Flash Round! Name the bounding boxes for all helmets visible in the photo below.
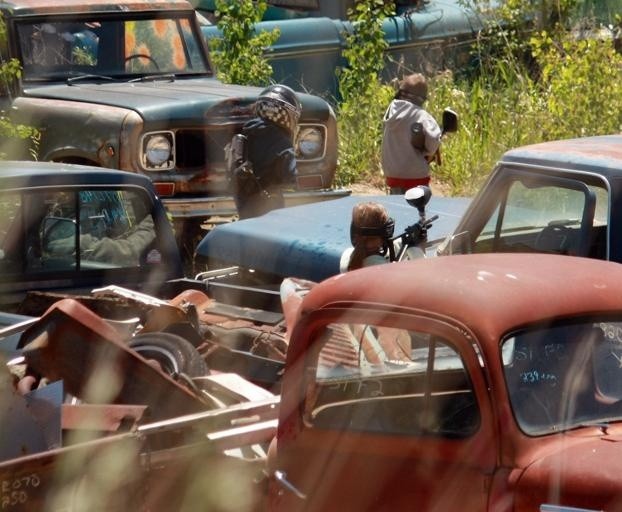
[259,85,303,129]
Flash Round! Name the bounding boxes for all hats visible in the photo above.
[399,72,428,100]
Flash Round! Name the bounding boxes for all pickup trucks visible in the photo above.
[0,3,527,306]
[163,134,622,310]
[1,251,622,512]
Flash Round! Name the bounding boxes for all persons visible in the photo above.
[27,191,173,266]
[381,72,444,194]
[338,202,429,378]
[226,85,303,220]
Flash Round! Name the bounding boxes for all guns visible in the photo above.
[410,108,458,166]
[384,185,438,262]
